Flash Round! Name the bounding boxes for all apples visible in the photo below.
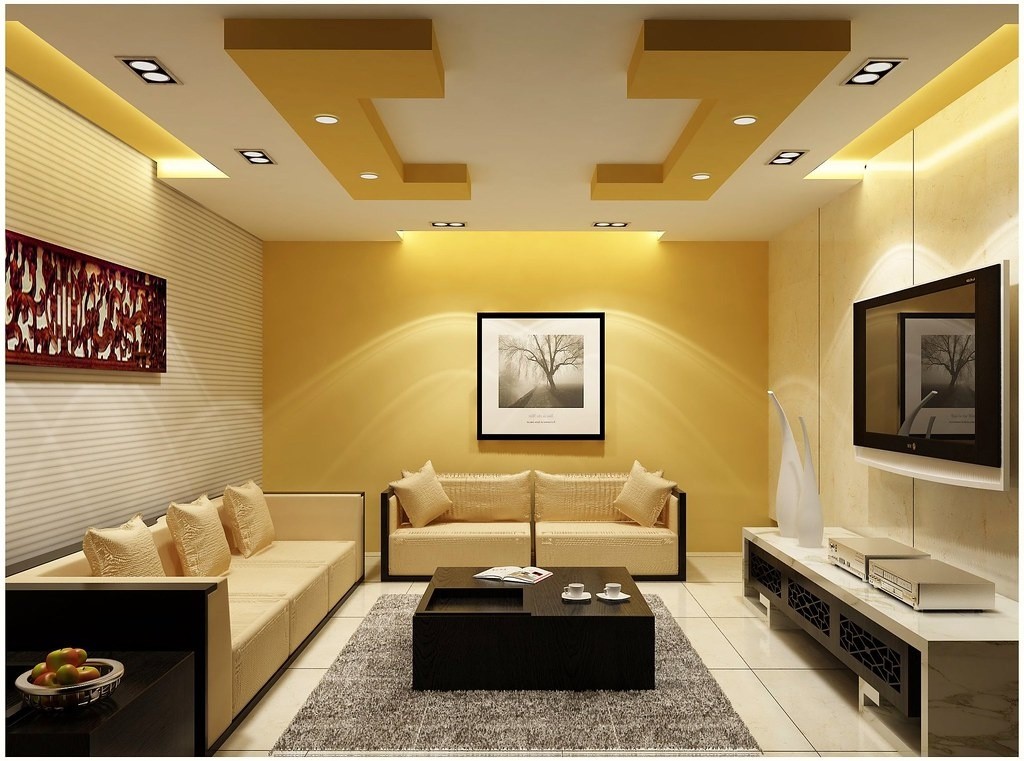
[29,646,100,704]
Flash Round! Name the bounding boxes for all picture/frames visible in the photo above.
[898,312,976,440]
[477,312,606,441]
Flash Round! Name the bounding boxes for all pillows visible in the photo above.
[613,460,678,528]
[166,489,232,577]
[388,460,452,528]
[222,478,274,558]
[82,512,166,577]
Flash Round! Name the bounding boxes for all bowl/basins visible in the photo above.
[15,658,125,709]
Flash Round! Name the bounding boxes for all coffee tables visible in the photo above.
[413,567,656,692]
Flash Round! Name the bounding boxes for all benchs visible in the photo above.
[380,470,687,582]
[5,491,366,757]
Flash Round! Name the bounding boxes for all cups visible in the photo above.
[564,583,584,598]
[604,583,622,597]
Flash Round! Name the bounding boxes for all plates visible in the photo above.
[561,591,591,600]
[595,592,632,601]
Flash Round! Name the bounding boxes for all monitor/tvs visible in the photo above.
[855,268,1001,468]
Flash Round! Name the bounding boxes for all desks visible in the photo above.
[6,650,195,756]
[741,526,1019,757]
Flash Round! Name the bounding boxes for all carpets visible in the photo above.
[267,593,763,756]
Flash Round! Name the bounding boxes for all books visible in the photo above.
[473,566,553,584]
